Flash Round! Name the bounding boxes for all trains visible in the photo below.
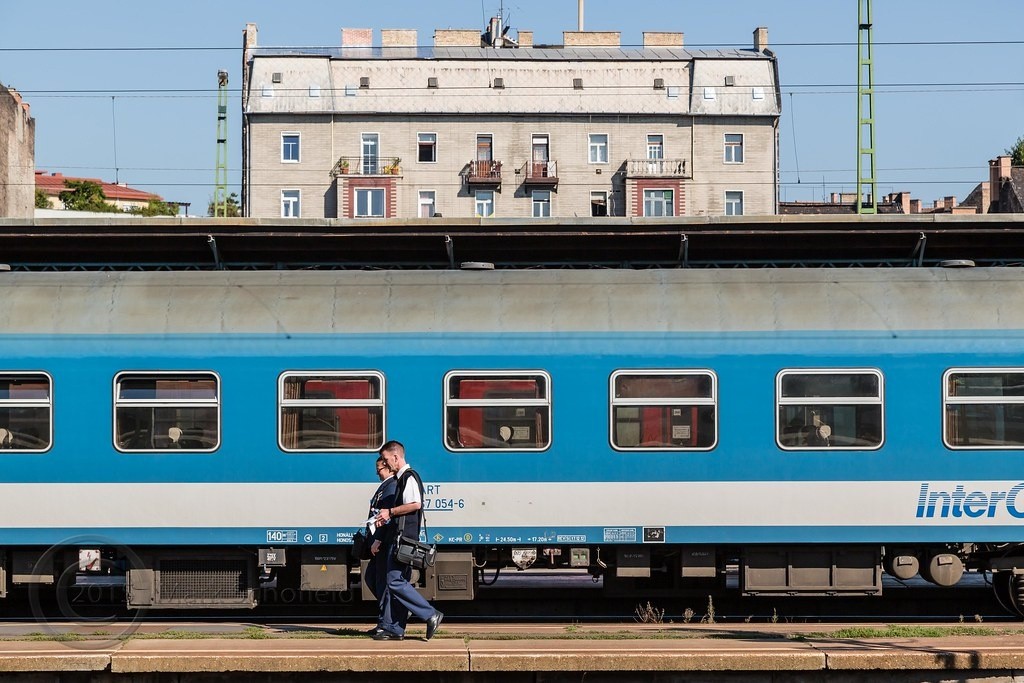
[0,209,1023,624]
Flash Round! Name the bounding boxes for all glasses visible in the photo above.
[376,466,388,474]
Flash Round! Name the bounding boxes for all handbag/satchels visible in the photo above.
[396,535,437,570]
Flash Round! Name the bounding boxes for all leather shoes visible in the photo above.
[426,610,444,640]
[372,631,404,641]
[406,609,412,620]
[367,626,384,636]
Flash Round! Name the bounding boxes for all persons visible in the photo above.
[363,441,443,641]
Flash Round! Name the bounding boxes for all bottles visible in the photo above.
[371,506,391,524]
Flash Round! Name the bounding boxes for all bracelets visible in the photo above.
[389,508,393,517]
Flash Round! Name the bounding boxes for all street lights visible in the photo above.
[213,69,230,218]
